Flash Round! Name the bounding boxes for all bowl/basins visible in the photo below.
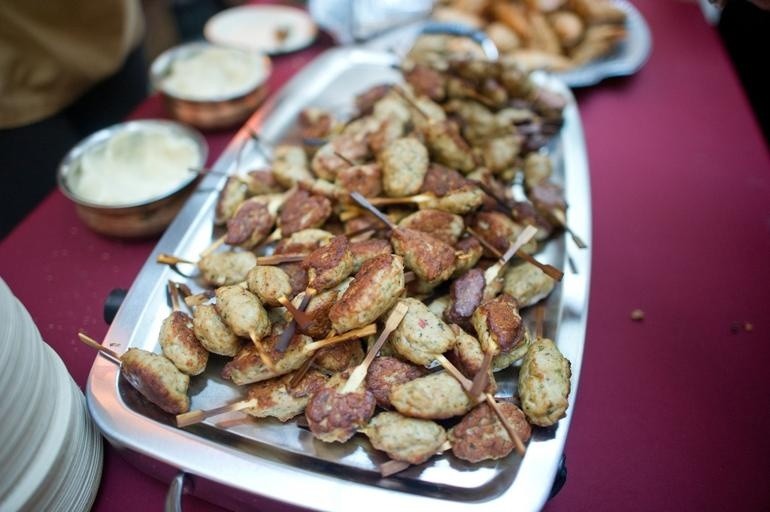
[149,39,273,128]
[57,118,212,242]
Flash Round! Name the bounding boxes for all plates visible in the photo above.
[1,277,104,510]
[201,1,322,51]
[428,1,655,90]
